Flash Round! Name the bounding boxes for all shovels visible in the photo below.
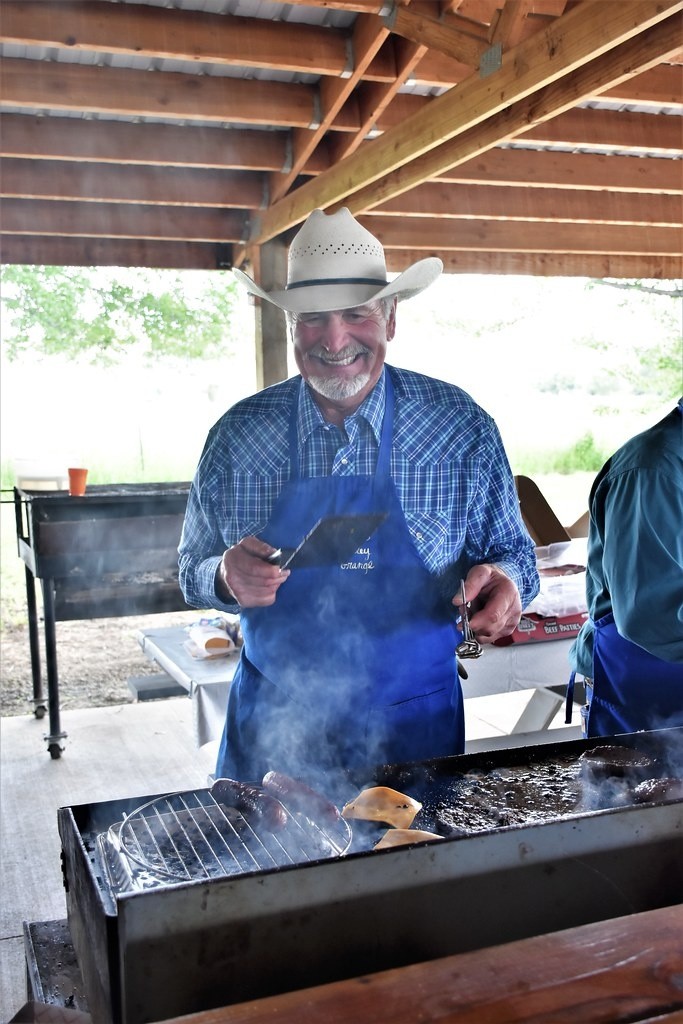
[269,512,389,571]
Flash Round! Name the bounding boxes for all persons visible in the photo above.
[177,208,541,778]
[570,397,683,737]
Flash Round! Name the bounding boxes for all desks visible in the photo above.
[136,618,587,788]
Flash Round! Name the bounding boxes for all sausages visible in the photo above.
[262,771,341,825]
[211,777,287,833]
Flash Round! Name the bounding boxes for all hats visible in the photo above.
[232,206,445,314]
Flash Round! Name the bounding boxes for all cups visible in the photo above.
[68,468,88,496]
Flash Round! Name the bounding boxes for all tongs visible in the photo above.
[455,579,484,659]
